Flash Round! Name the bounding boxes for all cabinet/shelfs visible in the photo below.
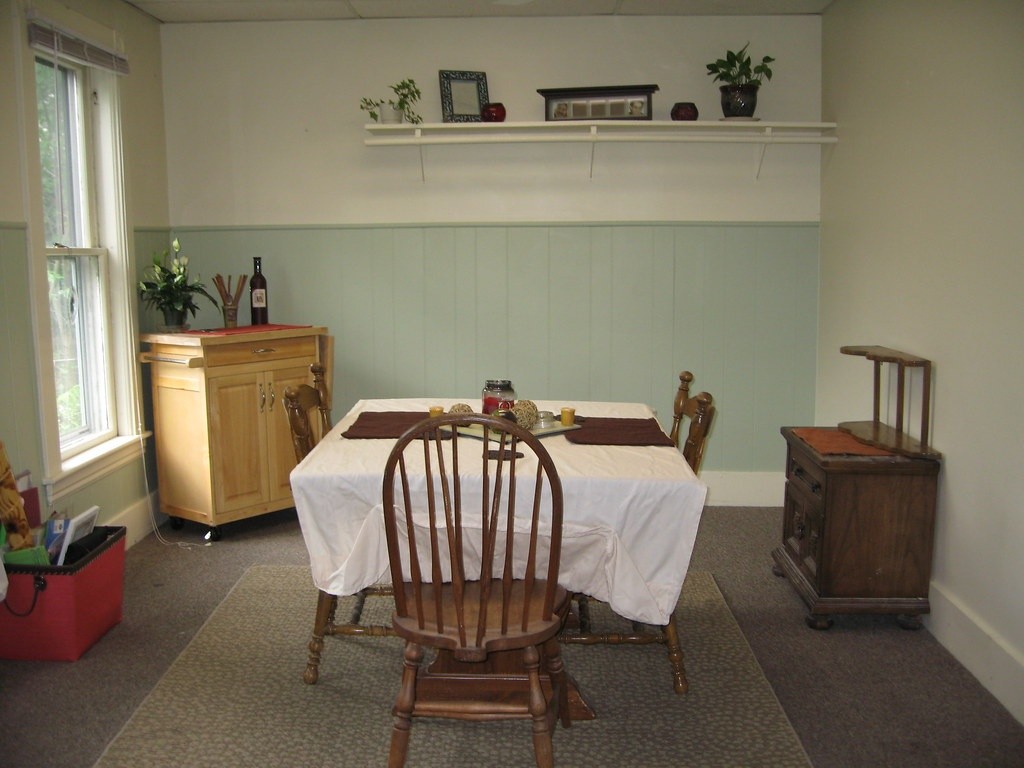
[139,324,333,539]
[838,345,942,461]
[772,426,940,629]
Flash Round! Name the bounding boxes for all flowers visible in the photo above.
[140,236,217,318]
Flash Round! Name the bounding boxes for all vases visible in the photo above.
[164,302,186,327]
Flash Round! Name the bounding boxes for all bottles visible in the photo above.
[249,256,268,325]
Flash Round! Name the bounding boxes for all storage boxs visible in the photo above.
[1,512,126,663]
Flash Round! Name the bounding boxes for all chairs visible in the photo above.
[283,364,402,687]
[382,411,571,768]
[556,370,715,694]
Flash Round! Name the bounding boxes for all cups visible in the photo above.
[429,406,443,418]
[561,407,575,426]
[222,306,238,329]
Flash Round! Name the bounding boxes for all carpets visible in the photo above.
[92,563,816,768]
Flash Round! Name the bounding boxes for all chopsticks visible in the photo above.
[212,273,247,307]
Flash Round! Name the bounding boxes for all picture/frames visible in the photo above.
[438,68,491,122]
[57,505,101,565]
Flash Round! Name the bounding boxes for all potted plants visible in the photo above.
[359,76,423,126]
[708,41,776,120]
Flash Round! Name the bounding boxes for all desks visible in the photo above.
[290,396,708,718]
